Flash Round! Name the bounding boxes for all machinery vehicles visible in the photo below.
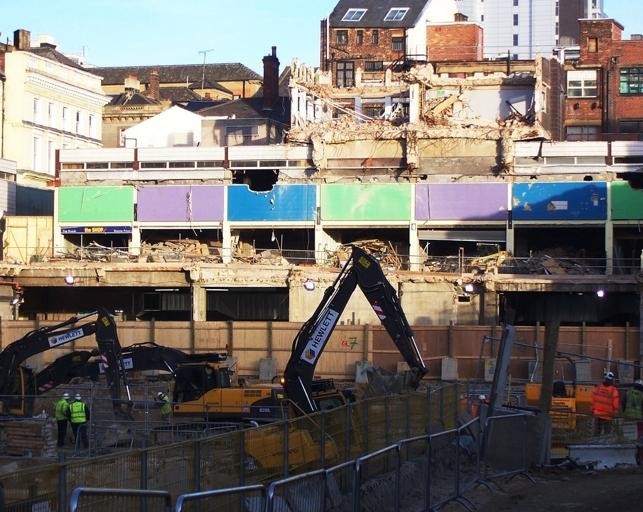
[0,246,289,424]
[234,244,430,480]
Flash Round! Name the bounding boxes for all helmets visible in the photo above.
[74,394,80,400]
[63,393,71,400]
[157,391,163,396]
[634,379,643,387]
[605,371,615,380]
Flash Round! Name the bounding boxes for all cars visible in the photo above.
[425,251,590,276]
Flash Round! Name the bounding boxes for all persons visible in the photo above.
[469,393,488,419]
[54,392,70,447]
[67,392,90,449]
[151,390,172,426]
[458,393,469,415]
[621,378,643,422]
[588,370,620,442]
